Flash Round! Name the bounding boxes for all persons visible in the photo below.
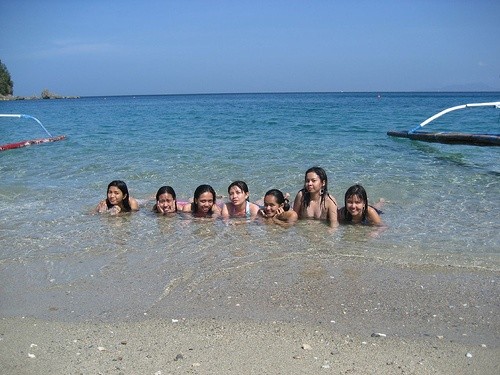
[153,185,187,219]
[337,184,387,239]
[293,166,338,236]
[257,188,299,228]
[95,180,156,216]
[221,180,264,226]
[183,184,222,223]
